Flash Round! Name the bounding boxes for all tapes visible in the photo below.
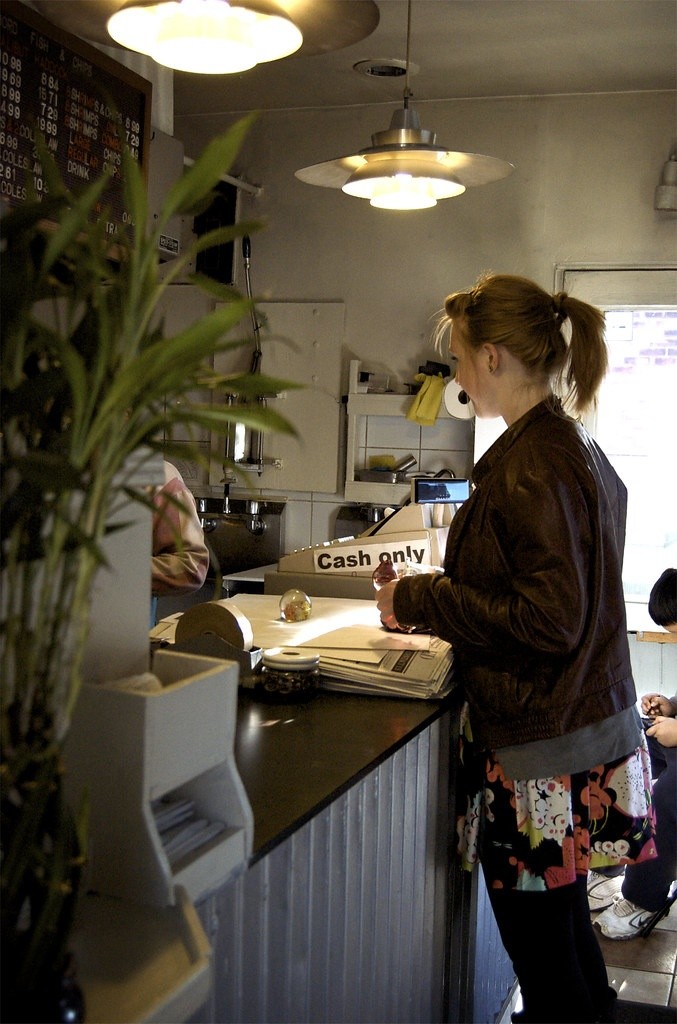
[173,599,254,652]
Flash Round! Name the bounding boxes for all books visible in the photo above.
[147,594,456,700]
[148,780,229,869]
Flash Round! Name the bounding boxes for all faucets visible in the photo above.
[195,498,218,532]
[246,500,267,536]
[220,477,239,515]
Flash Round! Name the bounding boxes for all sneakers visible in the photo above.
[591,892,665,941]
[587,869,628,911]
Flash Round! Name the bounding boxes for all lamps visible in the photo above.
[33,0,380,74]
[295,0,516,211]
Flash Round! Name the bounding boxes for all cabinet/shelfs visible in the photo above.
[345,360,473,505]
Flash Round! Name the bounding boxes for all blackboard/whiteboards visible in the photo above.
[1,0,153,265]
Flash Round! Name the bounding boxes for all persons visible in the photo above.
[375,276,657,1024]
[112,400,210,628]
[585,568,677,939]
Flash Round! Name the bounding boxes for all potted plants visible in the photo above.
[0,108,338,1024]
